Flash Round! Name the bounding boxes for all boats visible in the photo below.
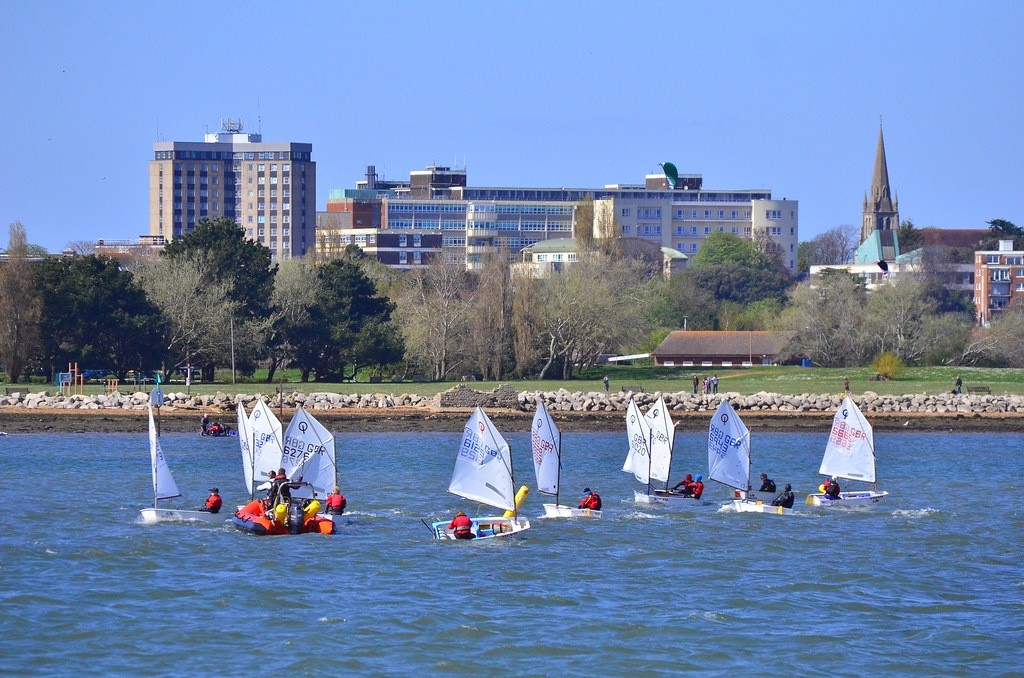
[232,479,337,536]
[200,422,239,437]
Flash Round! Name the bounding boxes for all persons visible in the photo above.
[448,511,476,540]
[693,375,699,394]
[325,486,346,515]
[603,375,609,391]
[256,468,303,520]
[759,474,776,492]
[578,488,601,510]
[200,488,222,513]
[844,378,849,392]
[672,474,704,500]
[201,414,208,434]
[702,374,719,393]
[955,376,962,394]
[772,484,794,508]
[824,478,840,500]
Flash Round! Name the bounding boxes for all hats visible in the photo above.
[695,475,702,480]
[583,488,591,495]
[686,474,692,480]
[209,488,219,493]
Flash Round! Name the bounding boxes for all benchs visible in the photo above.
[966,386,992,395]
[275,387,294,395]
[622,386,644,393]
[6,387,30,396]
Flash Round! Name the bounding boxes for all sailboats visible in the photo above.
[531,400,604,519]
[420,402,531,541]
[621,395,704,507]
[236,399,338,521]
[805,395,889,507]
[707,397,794,515]
[139,402,234,523]
